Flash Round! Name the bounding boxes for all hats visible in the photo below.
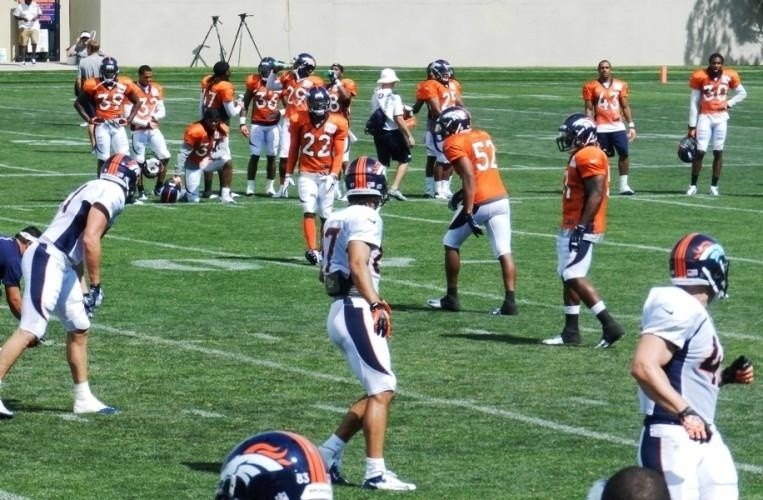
[203,107,223,122]
[14,224,38,243]
[213,61,230,75]
[376,68,400,83]
[80,32,90,38]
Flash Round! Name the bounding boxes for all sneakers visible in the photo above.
[449,176,454,196]
[387,185,407,201]
[441,180,452,199]
[272,185,289,198]
[362,469,416,491]
[126,196,143,204]
[423,177,435,199]
[318,460,352,485]
[305,249,320,265]
[427,296,460,311]
[489,301,519,316]
[151,180,165,195]
[686,184,697,195]
[202,192,219,199]
[230,191,239,198]
[0,397,13,416]
[138,185,149,200]
[265,190,277,196]
[538,329,582,345]
[216,195,238,204]
[431,181,448,199]
[590,324,626,349]
[619,186,634,194]
[709,185,719,195]
[246,191,256,195]
[73,396,122,414]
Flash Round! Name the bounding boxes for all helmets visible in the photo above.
[434,107,471,142]
[345,156,390,207]
[258,57,277,82]
[160,177,181,202]
[100,153,141,204]
[307,86,331,117]
[670,232,729,300]
[556,113,598,152]
[290,53,316,79]
[99,57,120,90]
[430,59,454,89]
[143,154,164,179]
[678,136,697,163]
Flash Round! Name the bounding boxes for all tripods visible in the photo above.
[219,46,227,63]
[227,13,264,70]
[189,16,226,69]
[190,46,210,69]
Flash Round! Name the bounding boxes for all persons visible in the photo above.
[74,57,147,205]
[413,59,464,200]
[167,109,236,203]
[544,113,627,350]
[0,225,45,346]
[283,86,349,266]
[73,40,105,153]
[13,0,43,65]
[200,61,244,204]
[66,32,106,67]
[266,53,325,196]
[588,467,674,500]
[366,68,415,201]
[582,59,636,195]
[325,64,357,201]
[426,108,518,315]
[124,65,170,200]
[0,155,142,417]
[689,52,747,195]
[240,57,282,196]
[319,158,418,491]
[631,232,755,499]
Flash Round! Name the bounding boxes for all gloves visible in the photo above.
[569,225,586,252]
[673,405,713,444]
[371,298,392,338]
[626,122,637,142]
[448,189,465,210]
[319,173,338,191]
[721,357,754,384]
[83,293,94,319]
[89,282,104,311]
[466,213,484,237]
[408,136,415,148]
[89,116,104,125]
[112,118,128,128]
[282,173,295,197]
